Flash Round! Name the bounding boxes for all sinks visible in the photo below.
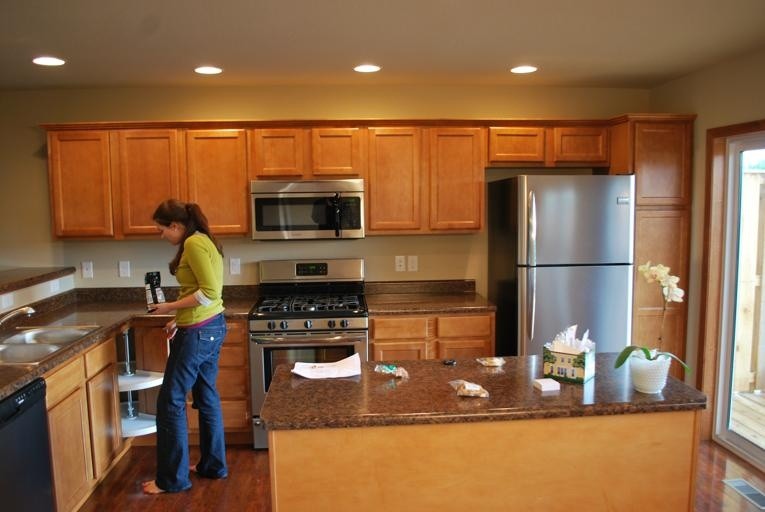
[2,324,104,344]
[0,345,66,366]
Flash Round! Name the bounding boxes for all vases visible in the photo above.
[629,352,671,395]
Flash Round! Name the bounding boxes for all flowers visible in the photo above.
[613,257,694,377]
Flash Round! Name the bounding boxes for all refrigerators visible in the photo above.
[485,171,636,356]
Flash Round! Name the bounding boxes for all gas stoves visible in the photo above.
[248,284,368,330]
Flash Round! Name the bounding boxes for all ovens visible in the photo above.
[247,331,368,451]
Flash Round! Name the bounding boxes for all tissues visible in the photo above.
[542,324,597,386]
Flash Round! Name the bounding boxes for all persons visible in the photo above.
[141,197,227,495]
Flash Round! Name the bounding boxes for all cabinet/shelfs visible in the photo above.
[243,120,362,178]
[601,112,697,382]
[39,119,252,242]
[370,312,496,362]
[134,320,252,446]
[43,337,124,512]
[354,119,487,236]
[487,119,608,168]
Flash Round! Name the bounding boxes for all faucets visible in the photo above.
[0,306,36,327]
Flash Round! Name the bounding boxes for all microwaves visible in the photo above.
[250,180,366,240]
